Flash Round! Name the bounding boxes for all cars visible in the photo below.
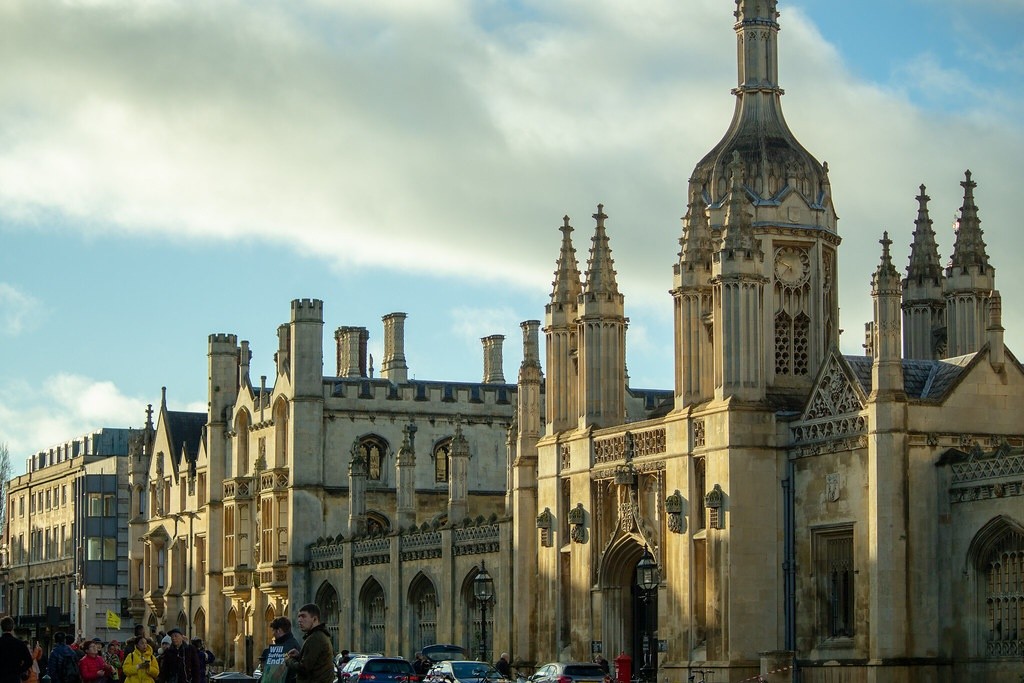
[524,662,616,683]
[332,644,511,683]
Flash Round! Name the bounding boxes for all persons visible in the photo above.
[594,655,610,675]
[496,653,512,681]
[0,616,127,683]
[260,616,301,658]
[338,649,351,666]
[284,603,335,683]
[120,625,215,683]
[411,652,425,674]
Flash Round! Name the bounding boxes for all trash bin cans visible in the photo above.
[757,650,795,683]
[208,671,259,683]
[614,655,632,683]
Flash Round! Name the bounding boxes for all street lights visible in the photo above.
[636,543,658,683]
[473,559,494,661]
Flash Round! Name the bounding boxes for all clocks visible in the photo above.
[773,245,811,289]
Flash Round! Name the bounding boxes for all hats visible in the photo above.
[257,649,270,659]
[167,628,182,635]
[110,640,120,647]
[92,638,105,648]
[158,634,173,655]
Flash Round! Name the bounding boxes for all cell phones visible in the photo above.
[145,661,150,663]
[37,641,39,648]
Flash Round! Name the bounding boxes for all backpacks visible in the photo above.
[54,648,79,682]
